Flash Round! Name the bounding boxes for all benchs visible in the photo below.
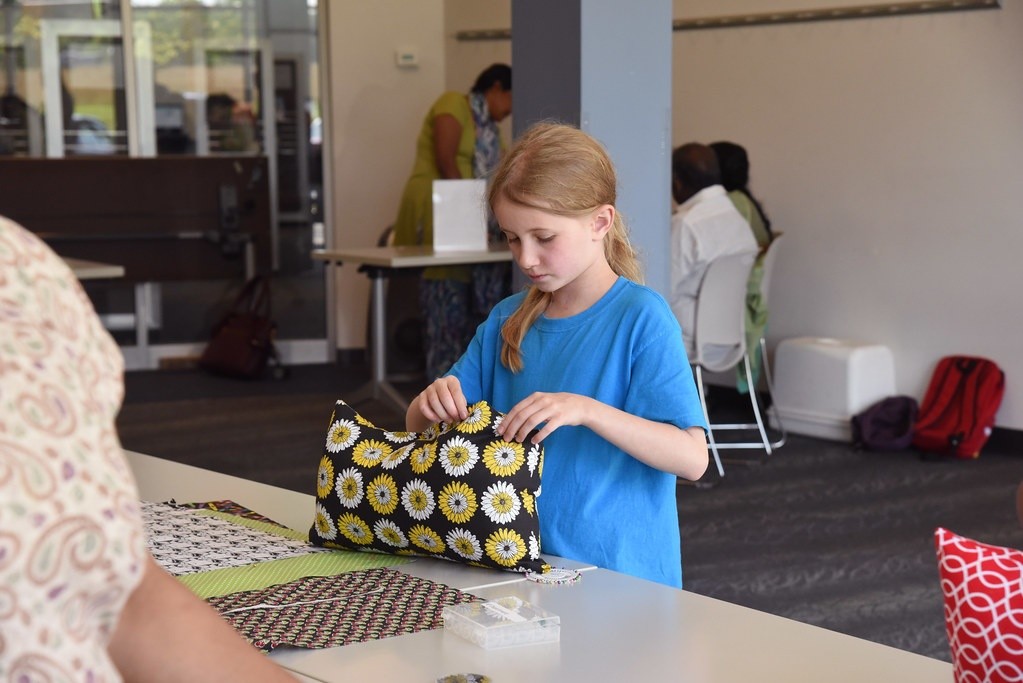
[0,155,266,370]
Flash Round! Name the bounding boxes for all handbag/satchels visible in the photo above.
[852,395,920,453]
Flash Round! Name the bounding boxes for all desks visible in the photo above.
[58,255,126,278]
[311,240,514,420]
[124,450,954,683]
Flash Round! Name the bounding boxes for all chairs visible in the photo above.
[682,230,787,489]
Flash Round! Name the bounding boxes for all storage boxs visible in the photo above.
[765,336,897,441]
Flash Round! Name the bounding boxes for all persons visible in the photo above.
[0,214,298,683]
[394,61,512,393]
[406,123,708,587]
[673,142,756,359]
[711,141,772,348]
[207,94,236,120]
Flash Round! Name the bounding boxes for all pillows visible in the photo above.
[308,399,550,575]
[934,527,1023,683]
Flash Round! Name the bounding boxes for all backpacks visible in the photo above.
[200,270,291,383]
[911,357,1004,459]
